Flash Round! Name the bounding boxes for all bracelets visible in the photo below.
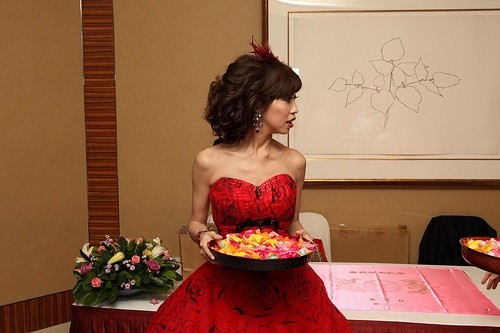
[196,231,209,247]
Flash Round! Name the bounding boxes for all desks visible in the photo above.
[69,262,500,333]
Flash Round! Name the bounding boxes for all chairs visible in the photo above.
[207,212,332,261]
[418,215,497,265]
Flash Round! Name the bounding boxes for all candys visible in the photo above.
[209,228,316,259]
[466,238,500,257]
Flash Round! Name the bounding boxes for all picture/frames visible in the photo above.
[261,0,500,191]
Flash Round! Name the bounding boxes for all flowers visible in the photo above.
[71,234,185,307]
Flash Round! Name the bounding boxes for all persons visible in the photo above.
[139,36,355,333]
[481,271,500,291]
